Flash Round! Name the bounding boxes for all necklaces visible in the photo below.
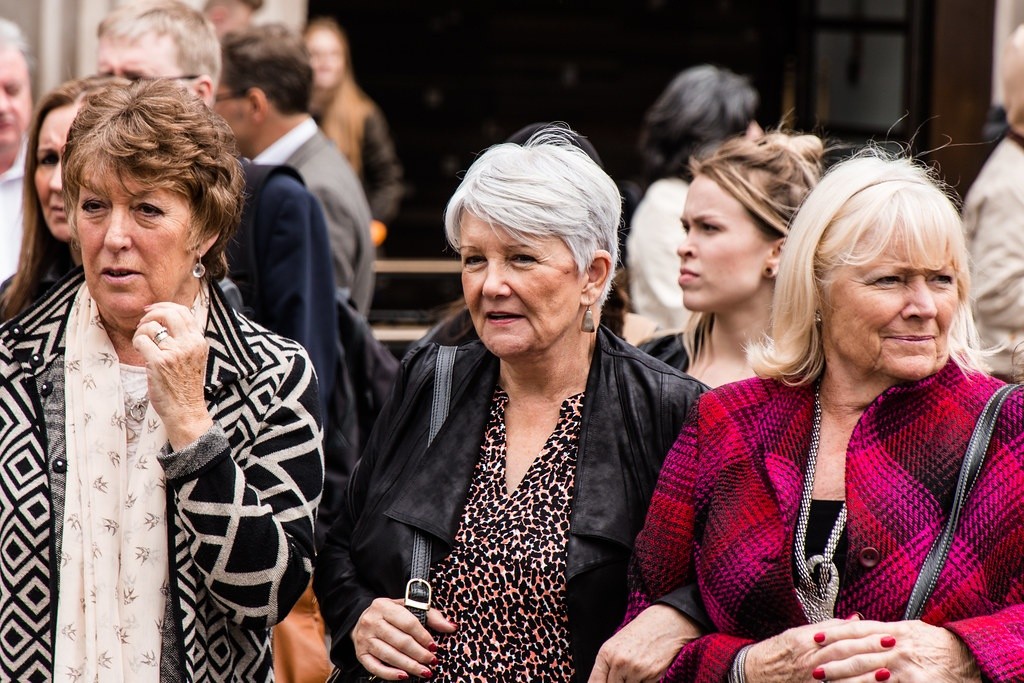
[793,380,848,625]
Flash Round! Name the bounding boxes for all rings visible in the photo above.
[154,327,168,343]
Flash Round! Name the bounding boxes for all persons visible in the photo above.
[313,122,713,683]
[632,120,825,392]
[0,0,408,683]
[961,28,1024,383]
[616,145,1023,683]
[617,63,767,339]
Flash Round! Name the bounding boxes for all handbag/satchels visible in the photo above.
[325,345,458,683]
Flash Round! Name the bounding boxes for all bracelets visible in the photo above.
[727,643,754,683]
